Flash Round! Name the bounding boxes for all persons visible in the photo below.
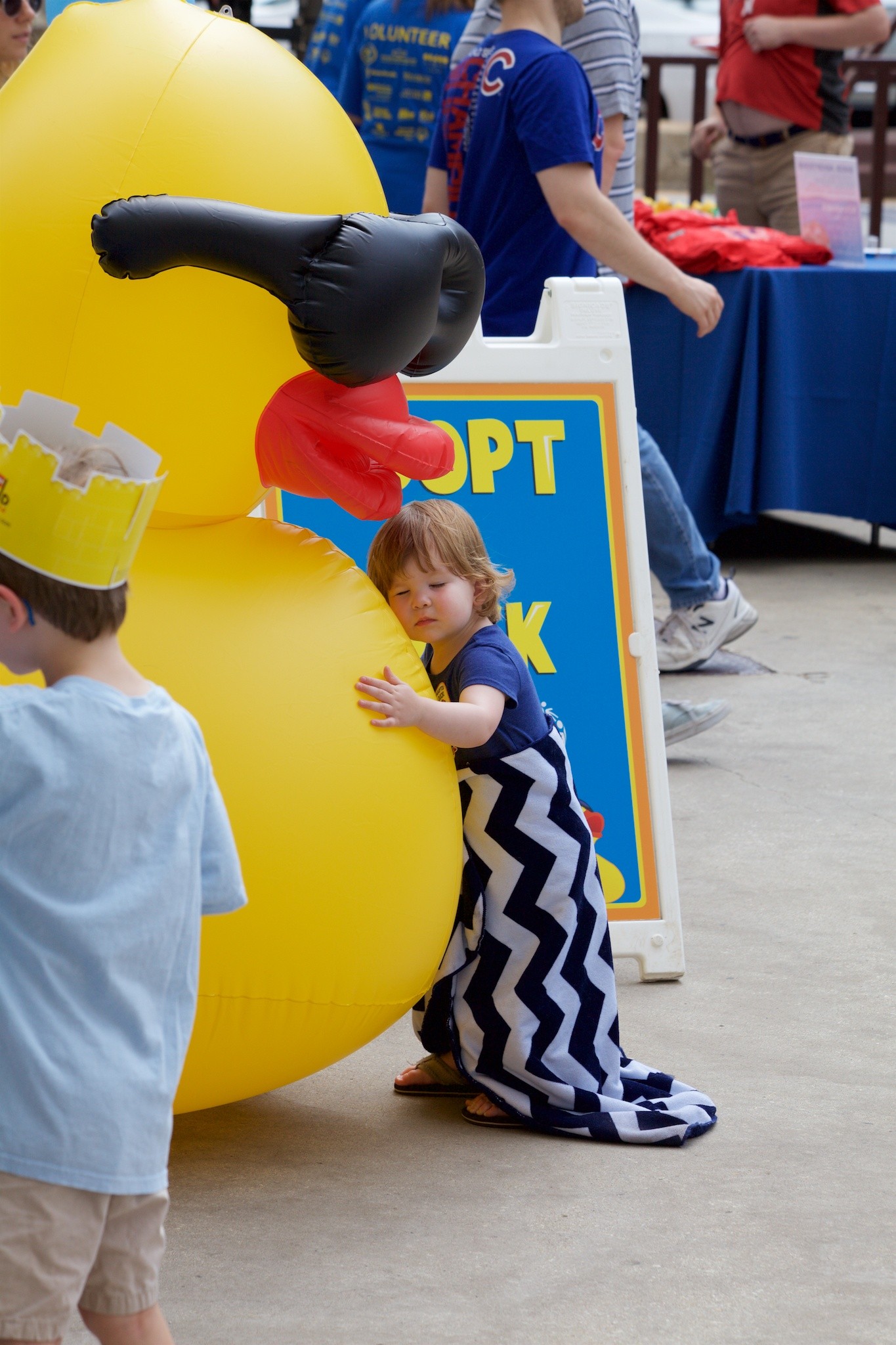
[419,0,733,746]
[448,0,760,671]
[0,391,249,1345]
[353,500,718,1145]
[689,0,890,235]
[1,0,472,265]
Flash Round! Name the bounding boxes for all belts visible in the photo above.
[725,120,805,148]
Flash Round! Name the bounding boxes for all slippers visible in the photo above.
[460,1092,521,1125]
[393,1053,479,1095]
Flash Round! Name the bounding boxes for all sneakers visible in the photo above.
[659,698,733,747]
[654,569,758,672]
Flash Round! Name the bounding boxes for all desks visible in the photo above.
[622,253,896,555]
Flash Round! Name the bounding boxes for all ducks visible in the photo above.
[0,1,465,1113]
